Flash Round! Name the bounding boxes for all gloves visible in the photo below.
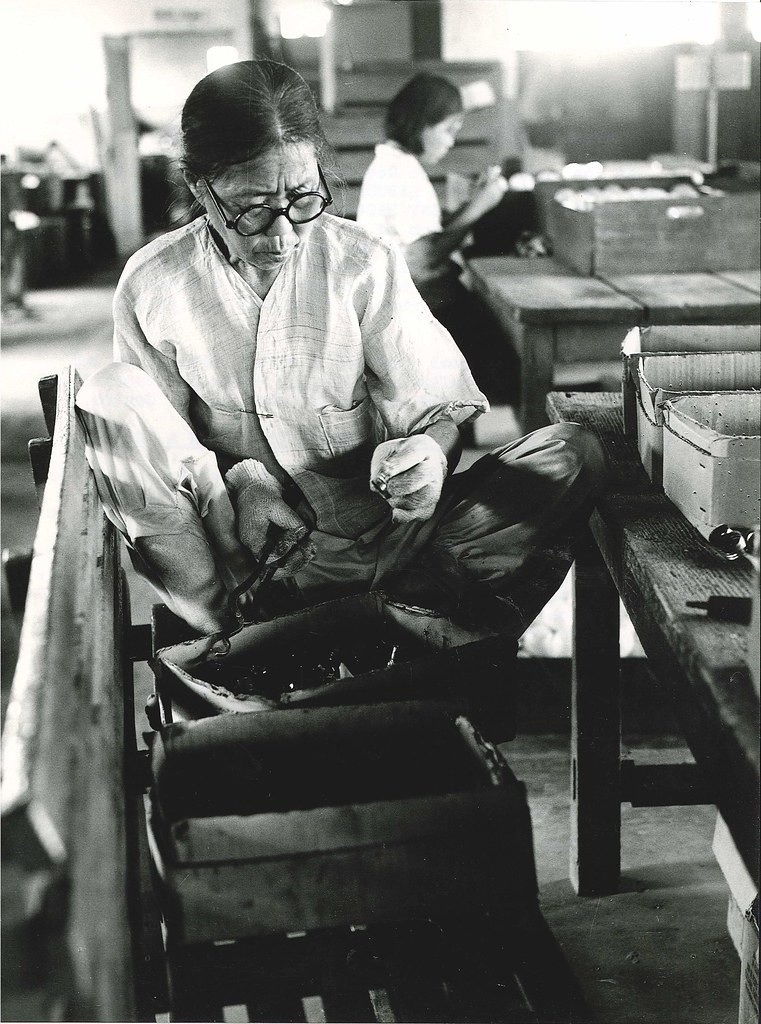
[370,433,450,523]
[224,459,317,581]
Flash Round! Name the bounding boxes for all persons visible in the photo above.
[109,61,605,741]
[356,71,518,410]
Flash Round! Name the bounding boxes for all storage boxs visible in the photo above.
[548,190,760,278]
[153,589,518,741]
[531,175,692,256]
[618,324,761,438]
[661,393,761,541]
[635,352,760,488]
[147,696,539,947]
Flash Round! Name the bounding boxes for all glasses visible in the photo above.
[203,162,335,237]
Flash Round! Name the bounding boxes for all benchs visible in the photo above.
[0,364,592,1023]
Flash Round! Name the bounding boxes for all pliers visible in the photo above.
[208,518,312,656]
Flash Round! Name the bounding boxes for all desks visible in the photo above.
[542,391,761,899]
[467,257,761,439]
